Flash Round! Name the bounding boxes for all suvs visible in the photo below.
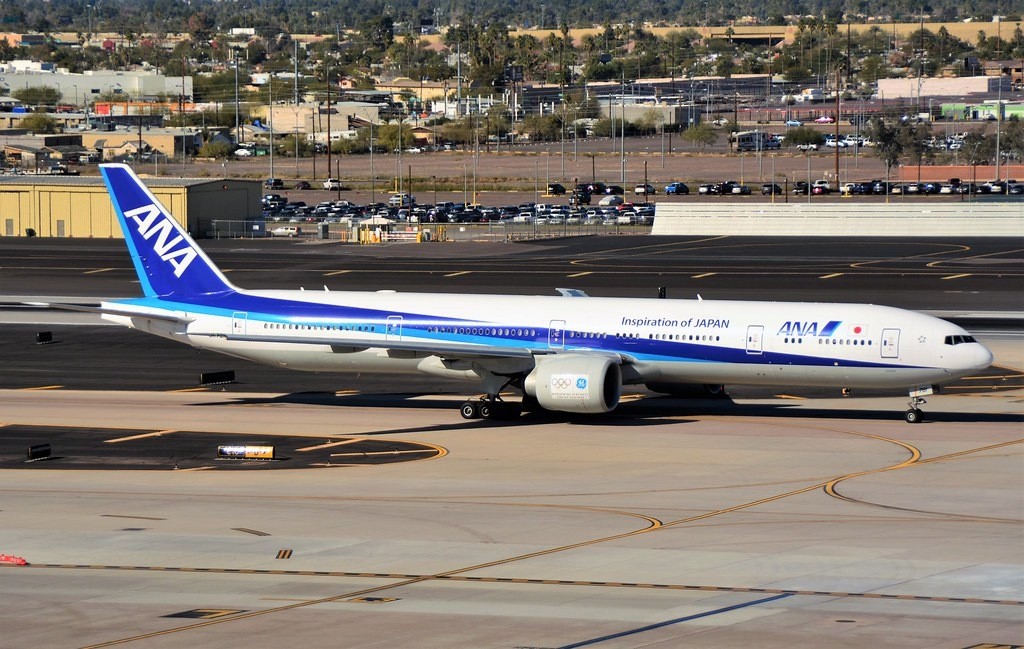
[849,113,871,125]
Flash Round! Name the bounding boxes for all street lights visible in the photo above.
[772,84,801,137]
[678,88,708,147]
[384,98,416,194]
[559,98,592,161]
[281,99,312,180]
[736,91,774,166]
[232,45,244,148]
[362,106,387,174]
[545,106,582,182]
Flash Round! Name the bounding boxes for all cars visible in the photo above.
[263,175,1020,231]
[786,120,803,127]
[901,115,918,124]
[814,116,834,125]
[723,128,1024,161]
[404,140,456,154]
[713,118,729,126]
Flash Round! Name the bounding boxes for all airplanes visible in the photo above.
[21,160,994,425]
